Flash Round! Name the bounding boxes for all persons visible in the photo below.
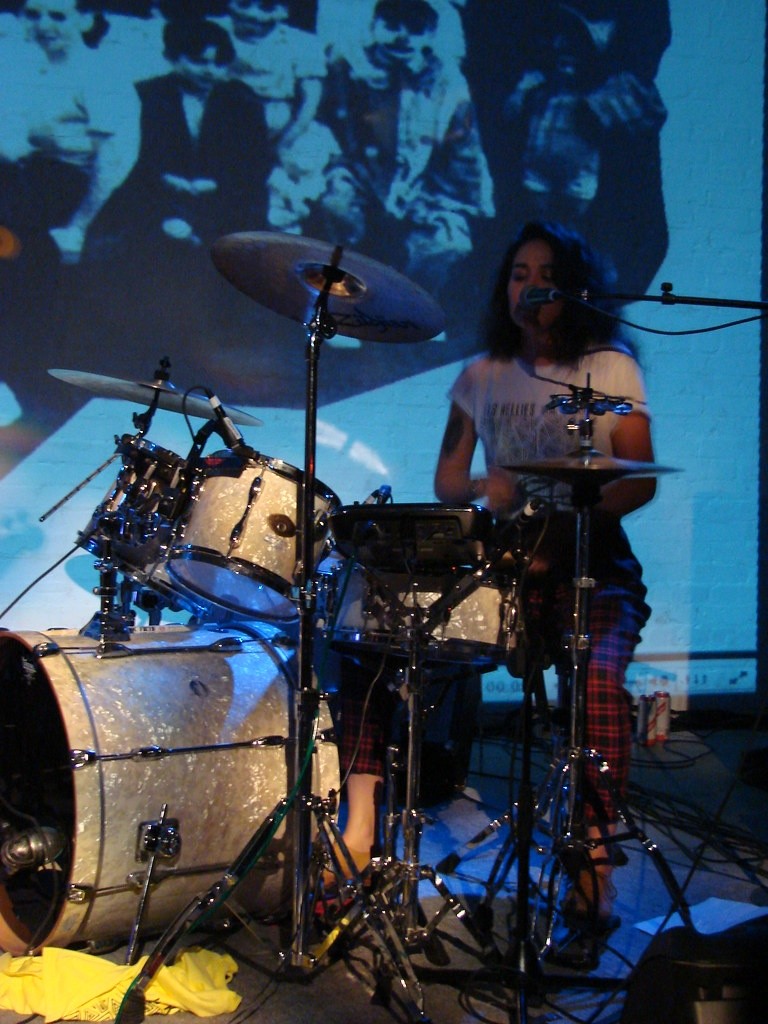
[0,1,672,407]
[304,220,655,919]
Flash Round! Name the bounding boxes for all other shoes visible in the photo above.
[560,870,616,920]
[322,845,378,887]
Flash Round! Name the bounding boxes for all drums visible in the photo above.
[330,548,511,668]
[161,445,344,622]
[72,433,182,594]
[1,619,345,948]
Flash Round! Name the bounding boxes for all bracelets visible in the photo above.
[468,479,480,497]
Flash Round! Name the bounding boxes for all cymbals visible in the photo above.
[210,228,444,346]
[497,449,685,486]
[46,366,263,427]
